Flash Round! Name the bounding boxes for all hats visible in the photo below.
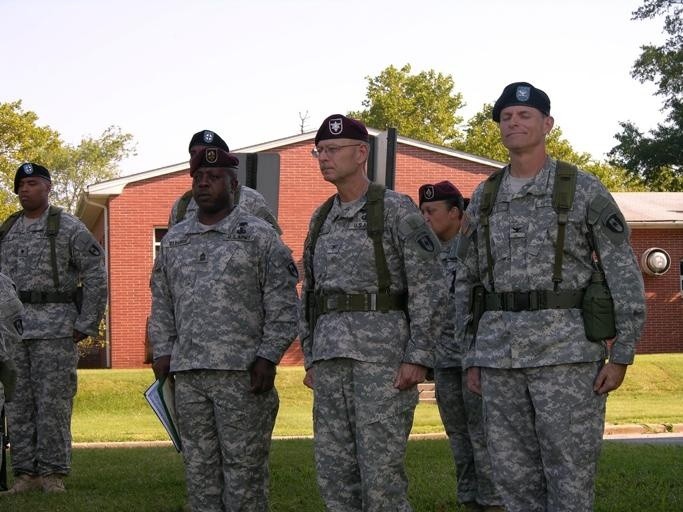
[191,149,240,172]
[15,163,52,191]
[316,114,368,141]
[188,130,228,150]
[419,181,463,203]
[493,82,550,119]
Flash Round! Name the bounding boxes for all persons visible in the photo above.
[417,178,488,510]
[452,81,646,512]
[150,145,298,512]
[0,161,108,496]
[167,129,284,237]
[0,272,28,470]
[296,112,443,512]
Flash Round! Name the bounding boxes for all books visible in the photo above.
[143,371,182,455]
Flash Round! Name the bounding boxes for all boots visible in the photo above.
[1,473,40,497]
[40,474,67,496]
